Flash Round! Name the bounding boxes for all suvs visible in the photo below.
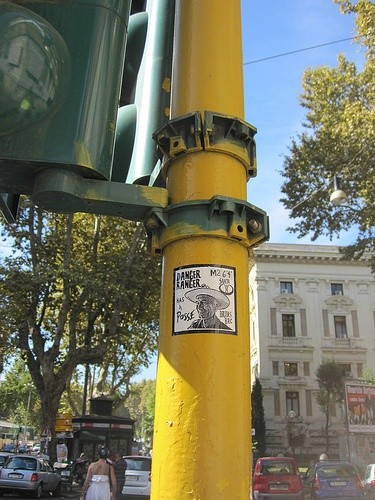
[116,455,151,500]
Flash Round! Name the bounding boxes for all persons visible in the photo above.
[80,447,117,500]
[111,451,127,497]
[76,453,87,486]
[2,443,33,455]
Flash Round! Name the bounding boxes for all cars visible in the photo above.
[33,444,41,451]
[311,459,375,500]
[253,457,306,500]
[0,451,62,499]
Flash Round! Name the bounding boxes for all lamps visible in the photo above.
[330,175,347,205]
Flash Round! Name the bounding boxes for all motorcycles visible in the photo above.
[66,451,98,487]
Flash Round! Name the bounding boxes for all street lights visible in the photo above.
[22,382,38,439]
